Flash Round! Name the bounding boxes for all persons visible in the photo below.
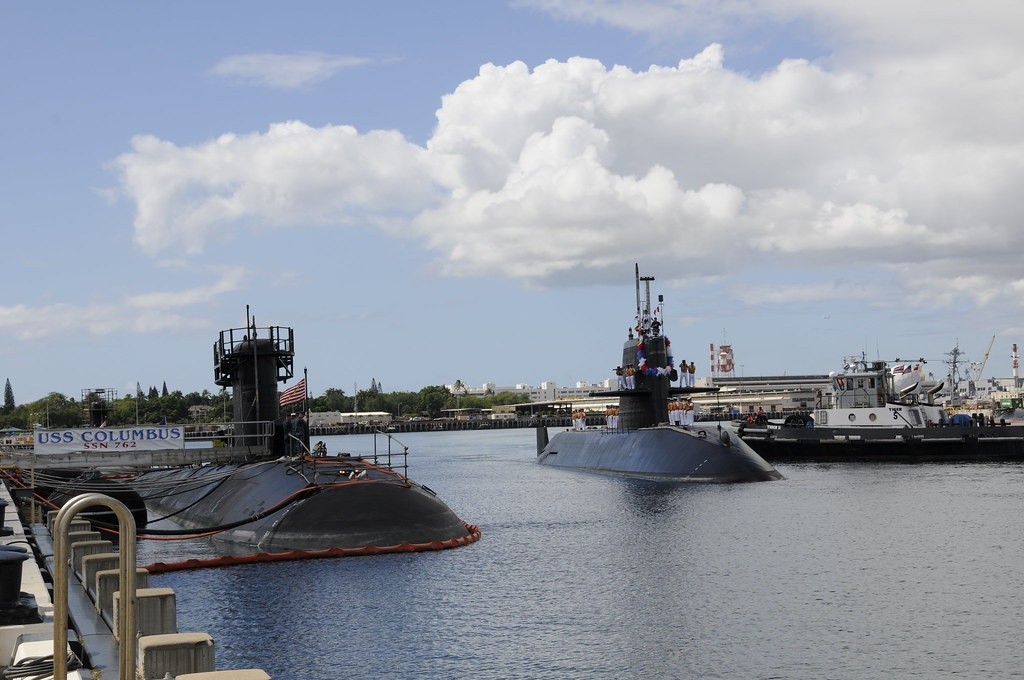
[747,415,753,424]
[625,364,631,390]
[757,407,764,414]
[295,411,308,456]
[651,317,661,336]
[667,397,694,429]
[630,363,636,389]
[291,413,298,458]
[679,360,689,388]
[605,405,620,430]
[642,310,655,334]
[613,367,628,391]
[572,408,586,431]
[282,414,293,457]
[688,362,696,388]
[314,441,327,458]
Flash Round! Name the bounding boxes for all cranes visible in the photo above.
[976,334,995,379]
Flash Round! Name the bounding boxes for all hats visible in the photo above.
[285,413,291,416]
[666,395,692,401]
[291,412,297,415]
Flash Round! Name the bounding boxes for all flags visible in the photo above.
[280,378,305,406]
[100,420,107,429]
[914,366,918,370]
[893,365,904,374]
[902,365,911,375]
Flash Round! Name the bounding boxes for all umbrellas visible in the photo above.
[1,426,25,433]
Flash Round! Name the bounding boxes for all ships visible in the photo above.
[729,332,1024,462]
[532,262,785,484]
[119,303,470,559]
